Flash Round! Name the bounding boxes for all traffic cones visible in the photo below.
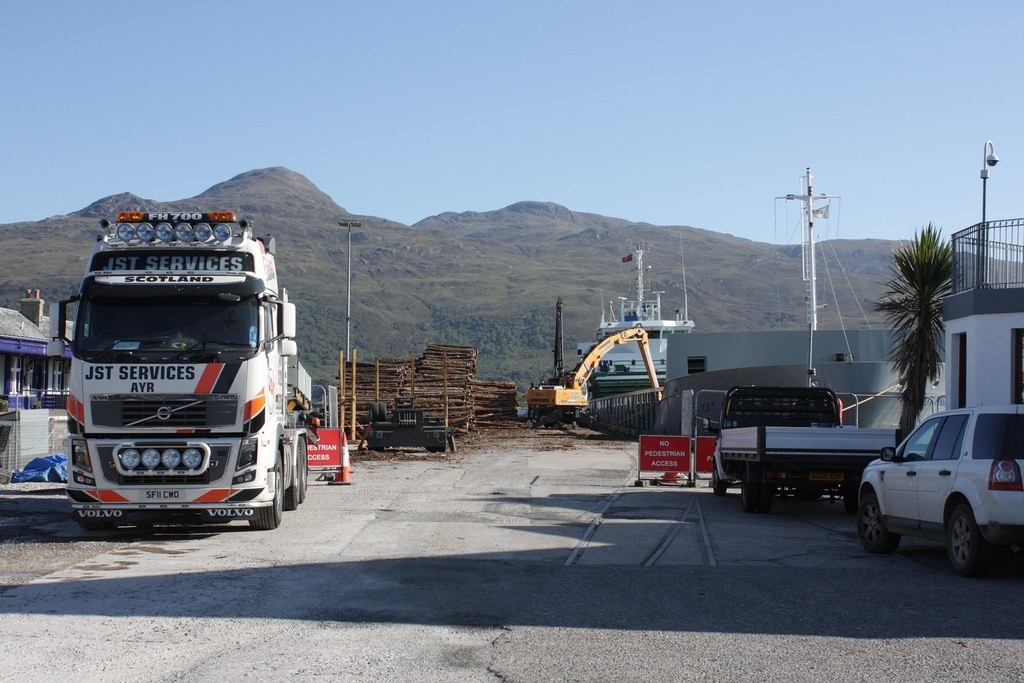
[328,432,355,485]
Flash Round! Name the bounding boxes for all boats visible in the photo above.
[577,238,696,429]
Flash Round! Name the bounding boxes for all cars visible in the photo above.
[856,404,1024,577]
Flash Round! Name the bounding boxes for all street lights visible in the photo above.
[338,219,362,443]
[979,140,1000,289]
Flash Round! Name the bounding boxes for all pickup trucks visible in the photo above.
[702,385,905,514]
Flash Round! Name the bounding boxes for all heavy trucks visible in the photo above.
[47,211,320,531]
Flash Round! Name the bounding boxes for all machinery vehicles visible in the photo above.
[527,328,663,428]
